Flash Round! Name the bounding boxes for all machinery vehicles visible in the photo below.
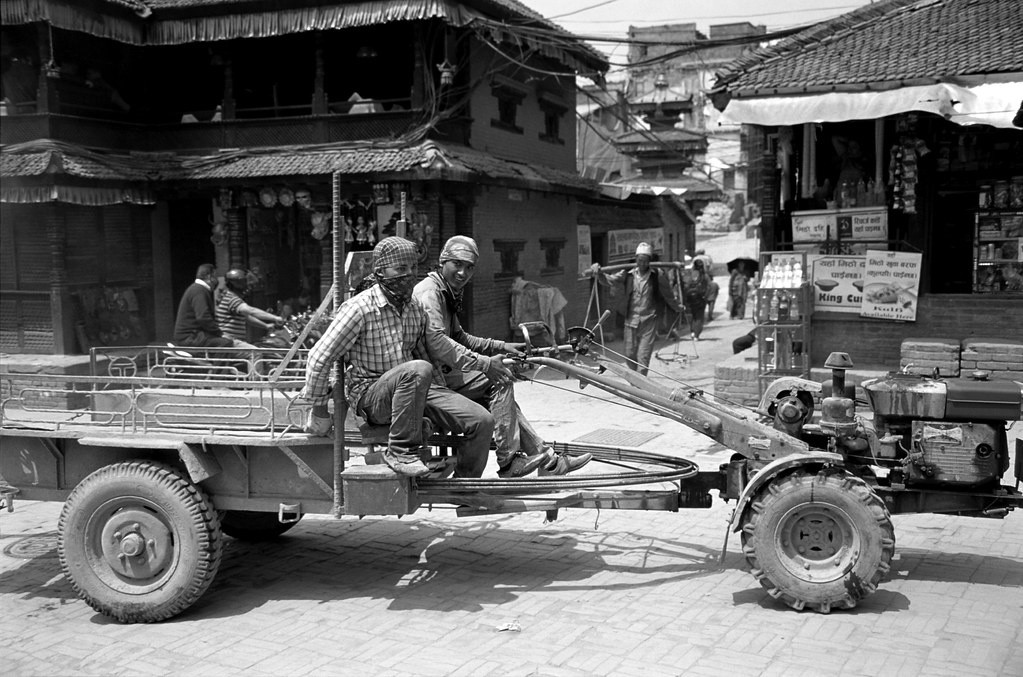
[0,171,1023,627]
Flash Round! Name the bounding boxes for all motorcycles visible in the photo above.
[162,305,320,391]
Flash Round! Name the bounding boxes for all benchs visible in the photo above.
[360,417,458,467]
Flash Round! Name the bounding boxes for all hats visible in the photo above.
[440,235,480,266]
[635,242,654,260]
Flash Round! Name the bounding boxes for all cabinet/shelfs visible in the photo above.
[792,205,887,256]
[756,251,814,402]
[973,212,1023,294]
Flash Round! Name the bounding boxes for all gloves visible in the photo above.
[307,413,335,439]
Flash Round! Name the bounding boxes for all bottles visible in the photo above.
[842,177,876,208]
[758,261,803,322]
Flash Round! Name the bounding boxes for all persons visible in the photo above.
[832,135,874,202]
[686,248,748,341]
[300,236,502,512]
[411,234,593,479]
[215,268,285,390]
[172,264,234,389]
[592,241,685,378]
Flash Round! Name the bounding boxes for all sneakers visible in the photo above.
[453,488,505,511]
[536,451,592,477]
[381,449,429,478]
[495,450,549,479]
